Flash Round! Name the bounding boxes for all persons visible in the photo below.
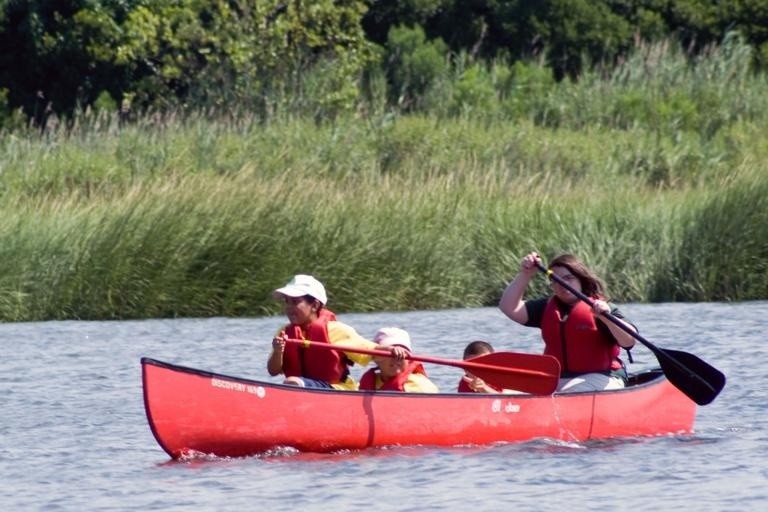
[455,340,504,395]
[357,326,440,393]
[266,274,413,391]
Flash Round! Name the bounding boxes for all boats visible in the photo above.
[141,357,696,460]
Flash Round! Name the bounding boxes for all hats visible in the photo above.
[373,327,413,353]
[272,274,327,306]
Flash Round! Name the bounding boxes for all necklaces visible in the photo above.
[498,250,640,393]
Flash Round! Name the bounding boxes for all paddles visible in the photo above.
[285,339,560,395]
[536,261,725,405]
